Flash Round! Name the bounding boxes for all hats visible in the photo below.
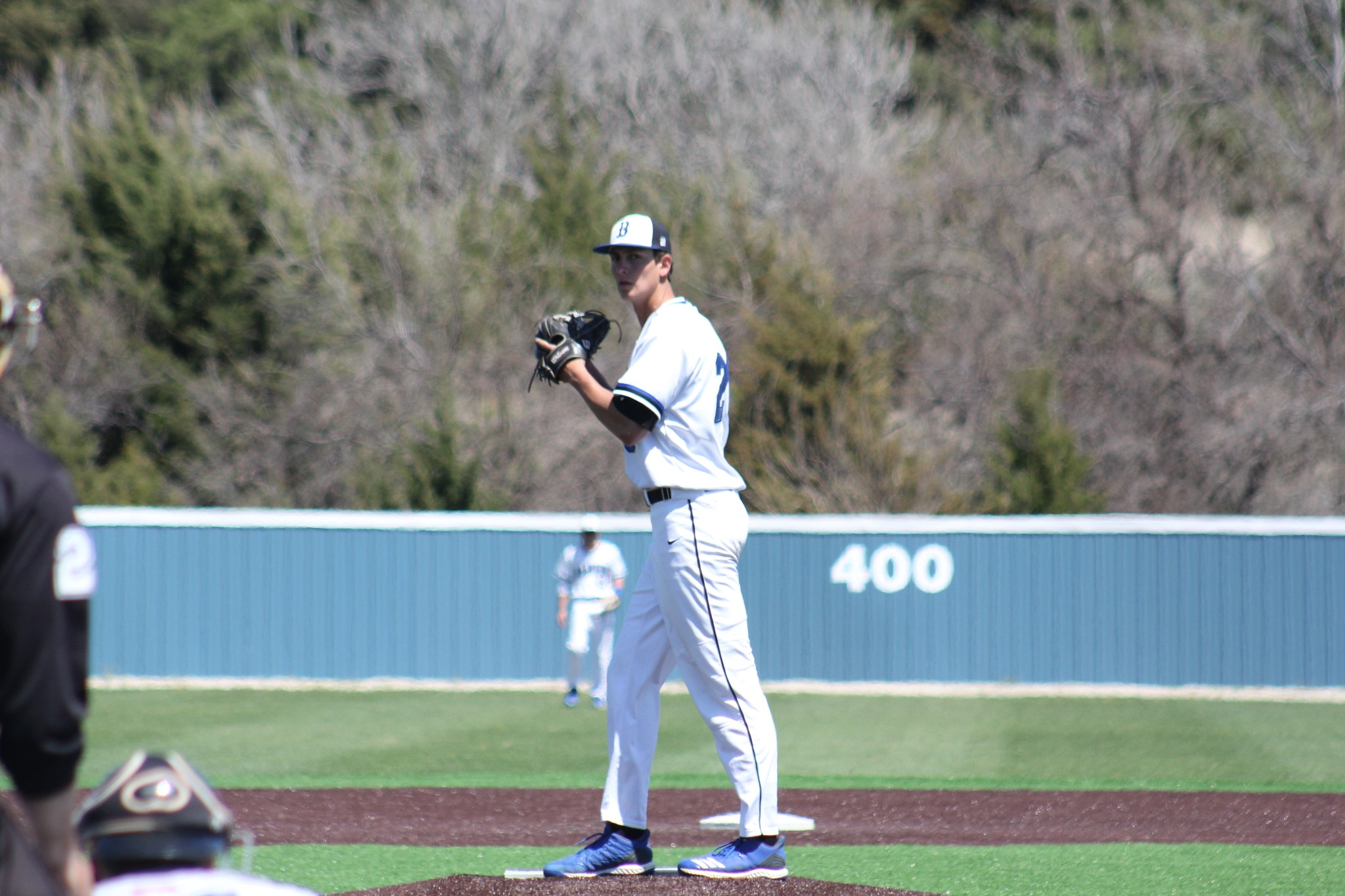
[593,214,671,254]
[580,515,601,533]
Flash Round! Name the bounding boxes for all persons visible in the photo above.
[558,524,626,707]
[73,746,317,896]
[0,269,94,896]
[533,213,790,880]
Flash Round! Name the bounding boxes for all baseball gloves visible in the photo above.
[534,310,610,386]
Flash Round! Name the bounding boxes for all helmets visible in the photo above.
[0,268,15,375]
[72,748,231,859]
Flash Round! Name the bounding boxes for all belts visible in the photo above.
[644,488,671,507]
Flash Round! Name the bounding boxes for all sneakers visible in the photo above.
[543,829,655,880]
[677,835,789,880]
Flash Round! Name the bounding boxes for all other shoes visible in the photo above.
[564,689,579,707]
[592,697,605,707]
